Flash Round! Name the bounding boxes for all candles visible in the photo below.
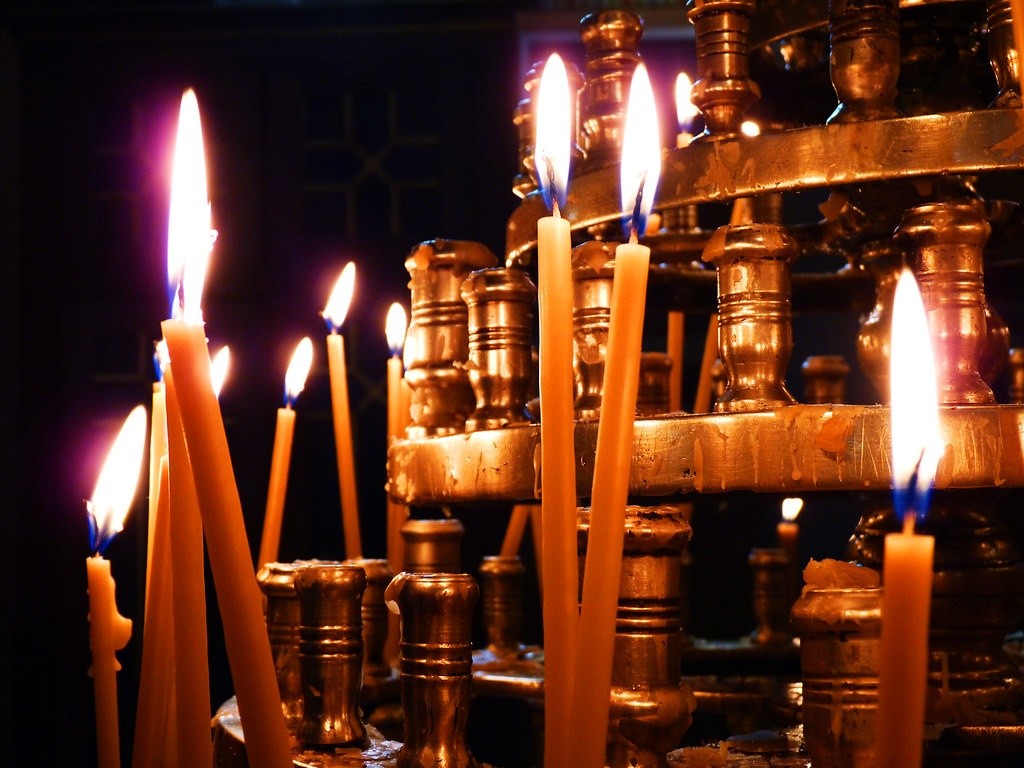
[531,54,578,768]
[382,300,406,567]
[321,260,367,560]
[85,89,313,768]
[565,59,663,768]
[872,267,935,768]
[777,499,804,550]
